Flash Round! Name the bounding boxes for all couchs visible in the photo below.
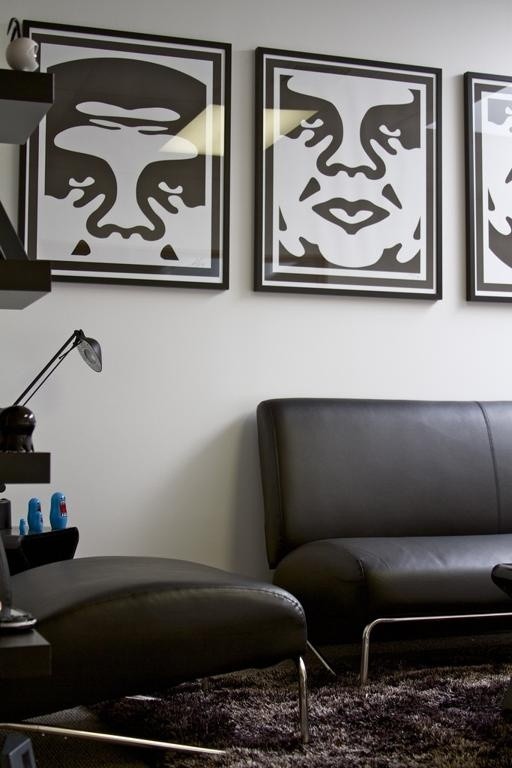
[255,399,510,684]
[0,506,313,752]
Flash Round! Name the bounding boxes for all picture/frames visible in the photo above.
[464,72,512,302]
[17,20,230,290]
[253,46,443,301]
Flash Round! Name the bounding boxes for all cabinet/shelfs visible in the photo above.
[0,452,78,578]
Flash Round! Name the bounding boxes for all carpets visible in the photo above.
[86,654,511,768]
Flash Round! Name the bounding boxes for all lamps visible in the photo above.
[1,331,103,452]
